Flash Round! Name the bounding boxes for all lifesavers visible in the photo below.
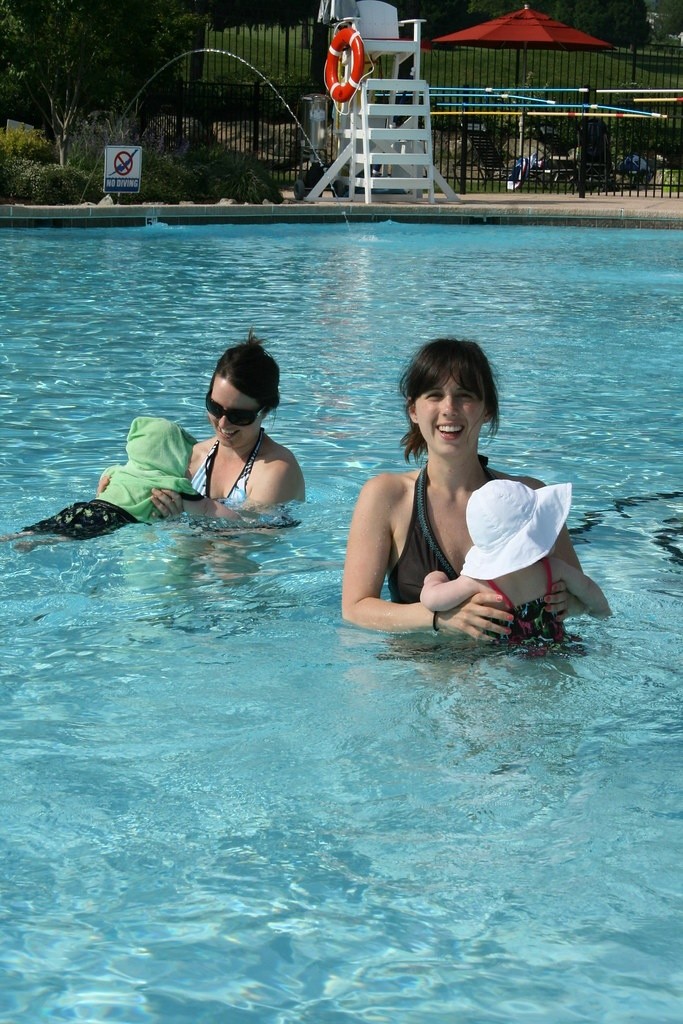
[326,28,365,102]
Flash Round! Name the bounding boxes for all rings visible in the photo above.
[166,513,171,519]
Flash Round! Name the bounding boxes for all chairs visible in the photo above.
[447,124,571,191]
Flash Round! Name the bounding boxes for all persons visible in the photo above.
[420,480,612,644]
[342,337,587,641]
[96,326,305,518]
[34,416,241,527]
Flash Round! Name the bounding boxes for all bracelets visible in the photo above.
[432,611,439,631]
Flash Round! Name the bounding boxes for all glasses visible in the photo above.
[205,391,266,426]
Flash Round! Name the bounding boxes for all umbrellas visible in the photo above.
[432,4,617,157]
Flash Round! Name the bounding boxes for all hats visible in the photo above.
[461,479,574,580]
